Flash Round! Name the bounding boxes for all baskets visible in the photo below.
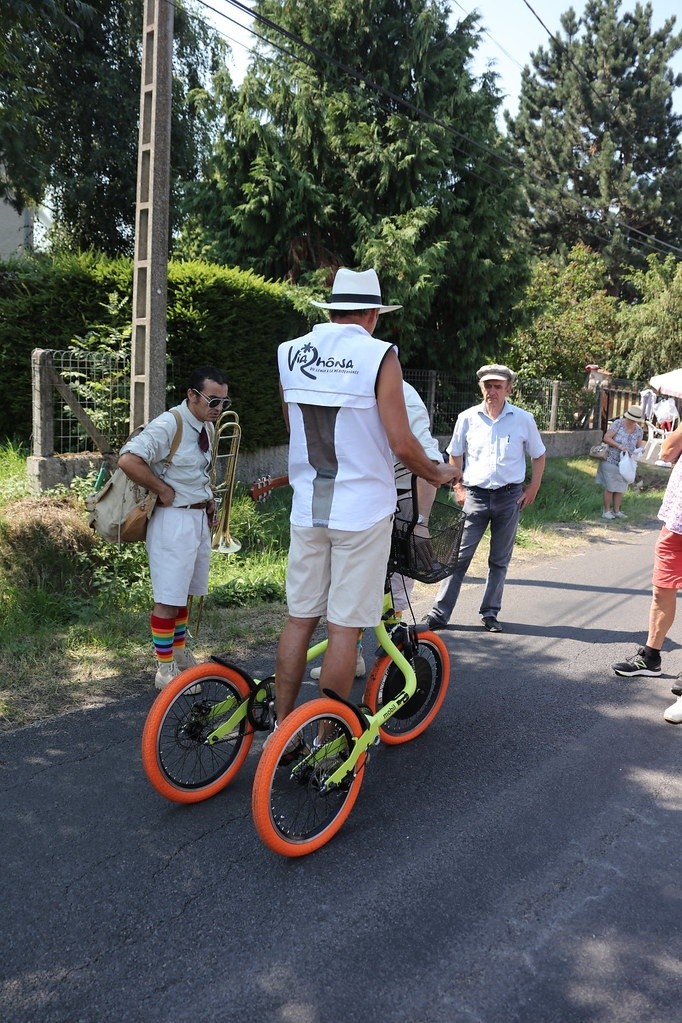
[390,498,467,584]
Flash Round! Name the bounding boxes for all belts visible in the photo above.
[176,503,206,509]
[468,484,513,494]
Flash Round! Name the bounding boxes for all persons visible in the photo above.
[422,364,546,631]
[612,417,682,723]
[263,268,462,766]
[309,376,444,706]
[116,367,230,695]
[595,405,645,519]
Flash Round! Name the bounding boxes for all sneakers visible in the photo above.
[421,615,444,630]
[310,644,366,679]
[672,672,682,695]
[480,617,502,631]
[155,660,201,695]
[602,510,615,520]
[612,645,662,677]
[663,697,682,723]
[175,648,197,670]
[611,508,627,519]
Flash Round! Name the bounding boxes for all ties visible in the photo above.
[198,427,208,453]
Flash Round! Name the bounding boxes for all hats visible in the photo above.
[476,364,515,384]
[310,269,404,314]
[624,405,643,422]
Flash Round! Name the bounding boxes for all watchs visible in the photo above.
[417,514,429,523]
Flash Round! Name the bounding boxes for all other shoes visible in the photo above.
[262,721,303,756]
[308,735,348,769]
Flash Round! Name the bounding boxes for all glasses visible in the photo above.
[484,384,502,389]
[191,388,231,410]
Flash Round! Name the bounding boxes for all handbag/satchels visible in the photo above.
[620,450,636,483]
[589,419,622,460]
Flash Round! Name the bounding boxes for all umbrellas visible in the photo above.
[650,368,682,399]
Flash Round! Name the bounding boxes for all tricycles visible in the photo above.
[142,453,467,858]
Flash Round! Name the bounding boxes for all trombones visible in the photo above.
[207,411,242,554]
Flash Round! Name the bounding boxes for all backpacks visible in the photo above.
[91,410,184,543]
